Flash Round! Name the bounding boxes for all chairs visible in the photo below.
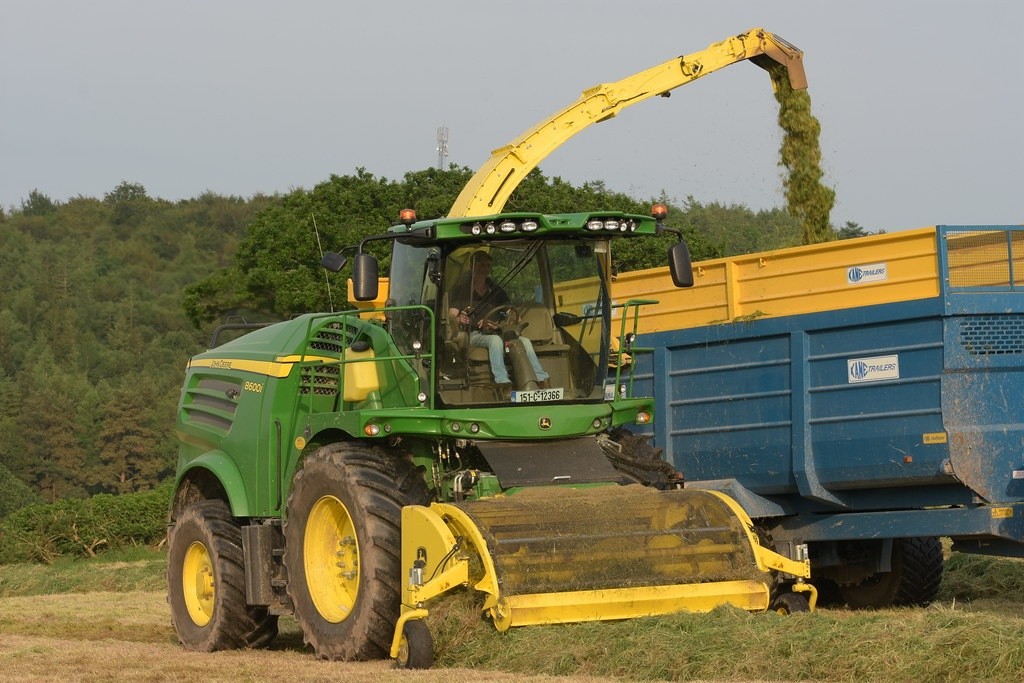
[442,257,507,361]
[520,301,570,353]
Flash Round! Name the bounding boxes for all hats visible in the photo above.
[470,251,493,261]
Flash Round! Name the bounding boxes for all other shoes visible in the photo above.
[497,383,512,401]
[540,377,552,389]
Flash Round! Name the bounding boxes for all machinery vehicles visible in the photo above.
[168,28,821,670]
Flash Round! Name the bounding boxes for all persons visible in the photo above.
[449,250,551,401]
